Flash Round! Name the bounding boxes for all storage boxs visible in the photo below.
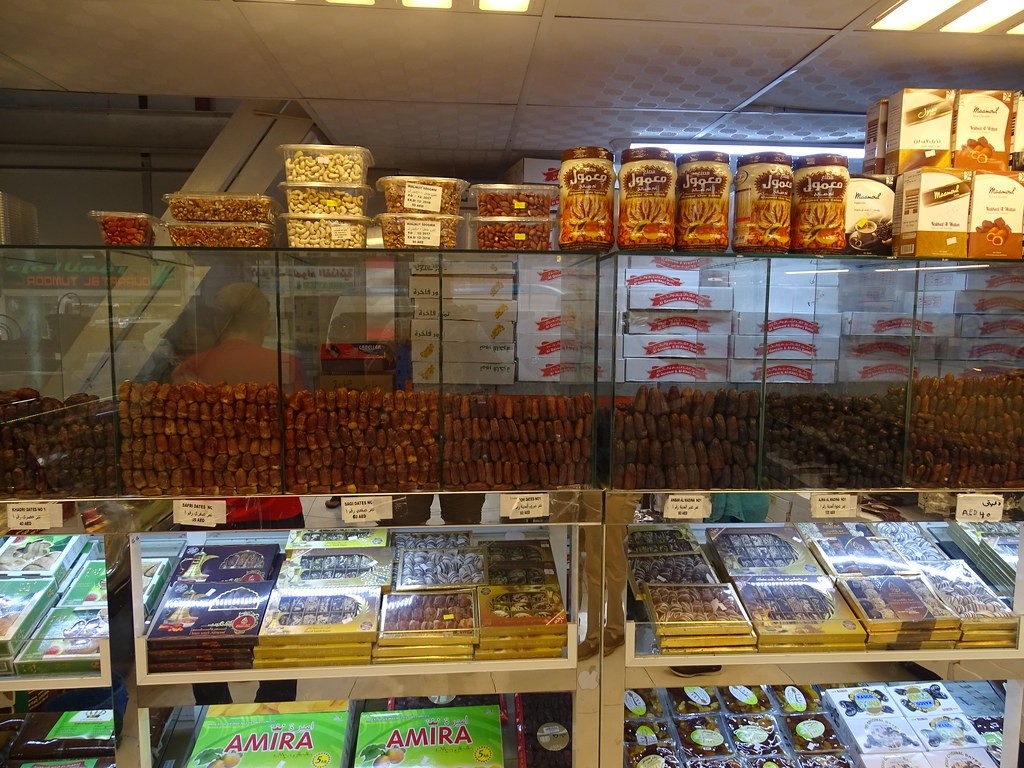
[394,252,1024,399]
[626,523,1020,654]
[186,701,505,767]
[822,681,997,768]
[0,527,569,676]
[317,343,396,395]
[843,89,1024,259]
[764,492,881,522]
[278,142,374,248]
[371,176,555,250]
[86,193,287,246]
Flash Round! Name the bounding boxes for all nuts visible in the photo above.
[285,151,366,248]
[168,197,278,247]
[475,192,552,252]
[380,180,463,250]
[97,215,155,247]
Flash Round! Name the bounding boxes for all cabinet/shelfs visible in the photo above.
[598,251,1024,768]
[1,242,600,768]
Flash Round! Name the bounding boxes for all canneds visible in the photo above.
[556,146,851,256]
